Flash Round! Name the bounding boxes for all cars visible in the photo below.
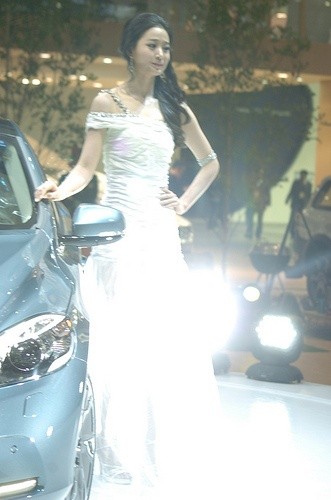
[0,116,128,500]
[296,176,331,315]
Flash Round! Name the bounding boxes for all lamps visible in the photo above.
[244,311,304,382]
[241,286,263,306]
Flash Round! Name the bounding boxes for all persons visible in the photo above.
[35,12,222,485]
[57,147,99,260]
[168,161,313,244]
